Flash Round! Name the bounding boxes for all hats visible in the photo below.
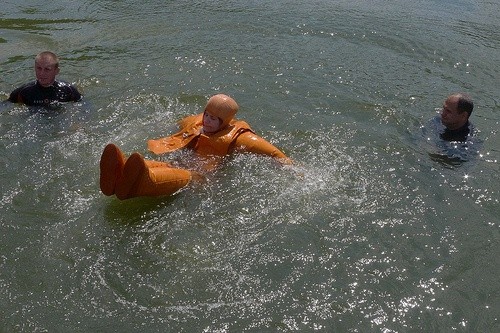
[202,93,239,126]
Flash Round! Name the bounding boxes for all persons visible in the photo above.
[0,51,85,115]
[426,93,482,154]
[99,93,304,201]
